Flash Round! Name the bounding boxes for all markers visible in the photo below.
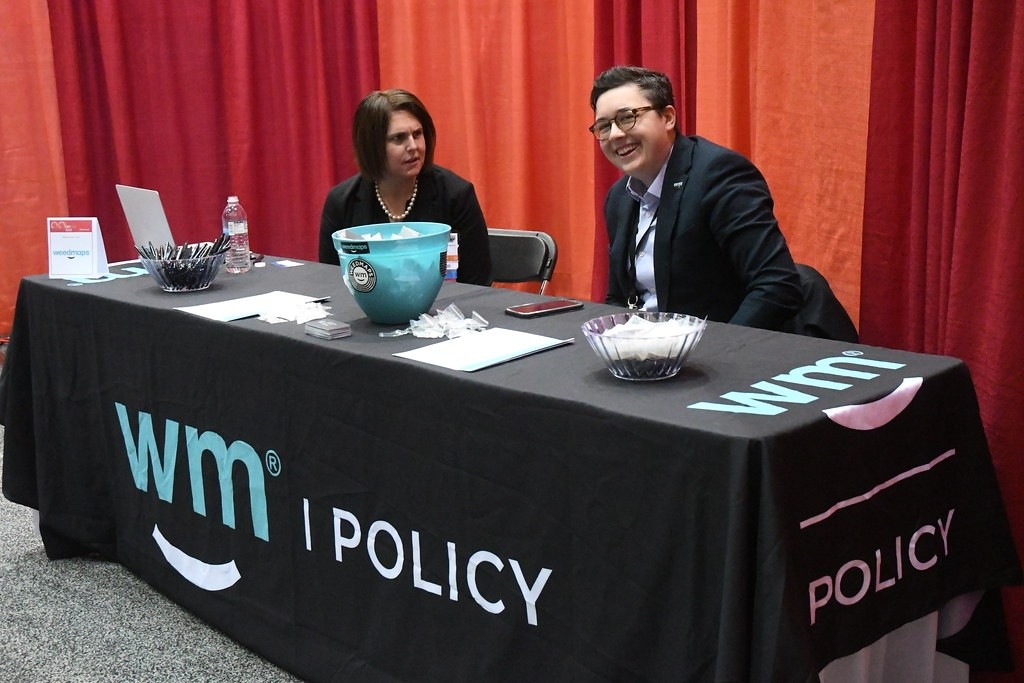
[253,253,264,265]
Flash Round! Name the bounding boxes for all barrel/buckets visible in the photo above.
[332,222,452,325]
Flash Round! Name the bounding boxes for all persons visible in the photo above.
[318,89,496,291]
[590,67,804,339]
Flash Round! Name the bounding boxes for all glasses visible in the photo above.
[589,103,665,142]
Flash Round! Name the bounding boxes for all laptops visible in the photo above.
[114,184,216,260]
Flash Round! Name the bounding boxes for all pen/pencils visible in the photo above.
[133,233,231,291]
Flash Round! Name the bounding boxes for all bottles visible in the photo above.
[222,196,251,274]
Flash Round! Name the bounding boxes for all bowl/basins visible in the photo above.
[581,311,706,381]
[136,245,226,292]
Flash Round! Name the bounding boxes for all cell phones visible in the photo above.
[505,298,583,319]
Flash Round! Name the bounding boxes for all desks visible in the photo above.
[3,247,1024,683]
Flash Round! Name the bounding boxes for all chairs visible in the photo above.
[487,229,559,296]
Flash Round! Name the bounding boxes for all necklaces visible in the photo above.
[623,196,663,310]
[373,177,421,220]
